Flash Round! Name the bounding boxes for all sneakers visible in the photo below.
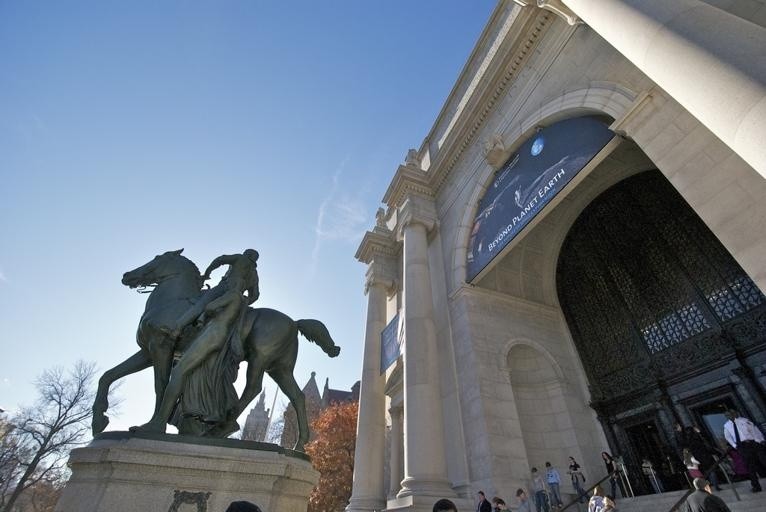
[552,503,563,509]
[750,486,762,492]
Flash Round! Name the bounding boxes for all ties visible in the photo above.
[732,421,742,446]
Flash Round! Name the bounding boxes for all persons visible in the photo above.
[683,428,723,491]
[588,486,616,512]
[724,409,766,491]
[684,478,731,512]
[478,456,591,512]
[601,452,628,499]
[642,457,665,494]
[226,501,262,512]
[432,499,458,512]
[129,249,259,433]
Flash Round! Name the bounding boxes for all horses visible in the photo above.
[90,246,341,454]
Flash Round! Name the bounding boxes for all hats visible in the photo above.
[692,477,707,489]
